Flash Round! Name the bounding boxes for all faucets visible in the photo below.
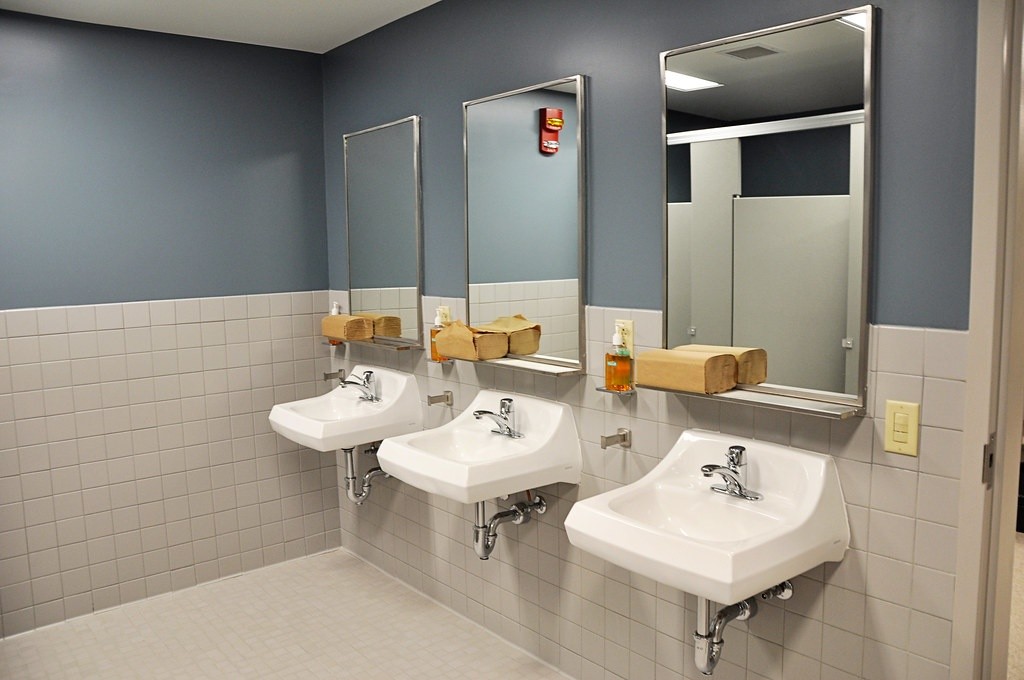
[473,398,524,440]
[339,368,375,400]
[700,444,765,504]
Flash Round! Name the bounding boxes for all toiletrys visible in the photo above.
[328,302,341,344]
[430,308,449,363]
[605,324,631,391]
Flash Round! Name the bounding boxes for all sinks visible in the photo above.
[563,427,852,605]
[268,364,424,453]
[376,389,583,505]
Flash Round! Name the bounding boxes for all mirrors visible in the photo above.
[463,74,590,371]
[659,3,879,409]
[341,115,425,347]
